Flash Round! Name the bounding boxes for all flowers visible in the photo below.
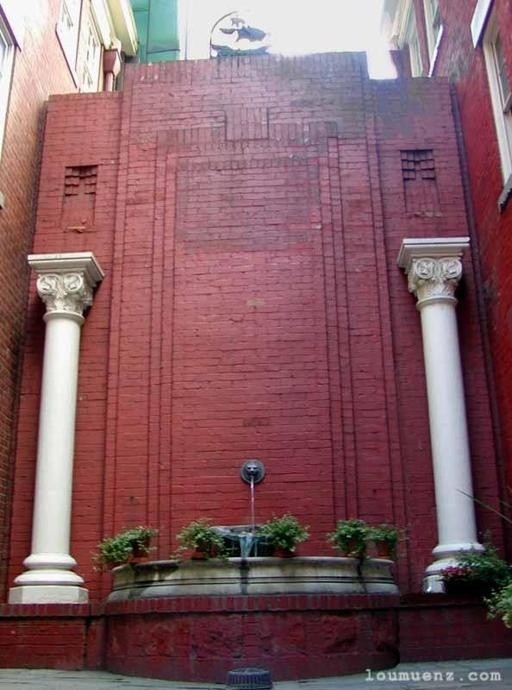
[441,564,469,578]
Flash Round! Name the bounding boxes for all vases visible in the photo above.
[439,579,469,594]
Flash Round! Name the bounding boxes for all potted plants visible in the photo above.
[90,532,133,567]
[261,512,313,557]
[170,517,237,572]
[124,524,160,565]
[326,519,370,559]
[371,523,404,559]
